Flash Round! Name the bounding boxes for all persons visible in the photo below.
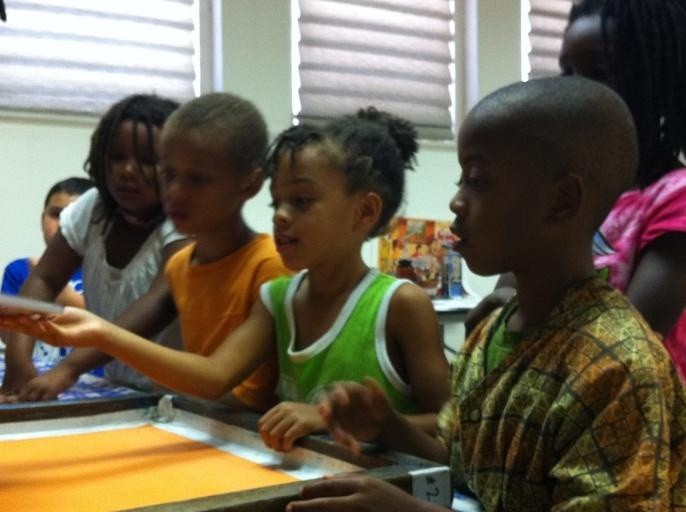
[3,179,96,379]
[19,93,294,414]
[287,73,684,512]
[2,109,451,453]
[3,95,176,400]
[558,0,686,382]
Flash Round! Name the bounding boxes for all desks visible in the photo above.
[0,350,453,511]
